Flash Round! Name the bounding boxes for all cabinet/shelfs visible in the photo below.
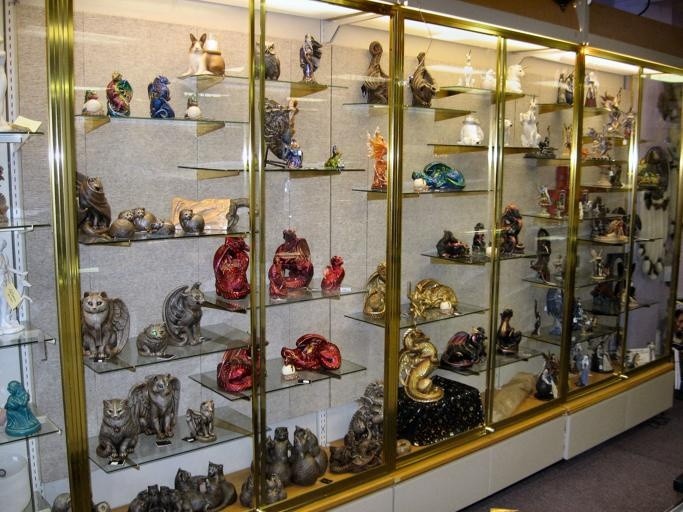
[1,1,683,512]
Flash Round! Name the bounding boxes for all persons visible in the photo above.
[0,239,33,336]
[436,231,469,259]
[472,224,486,255]
[4,381,38,429]
